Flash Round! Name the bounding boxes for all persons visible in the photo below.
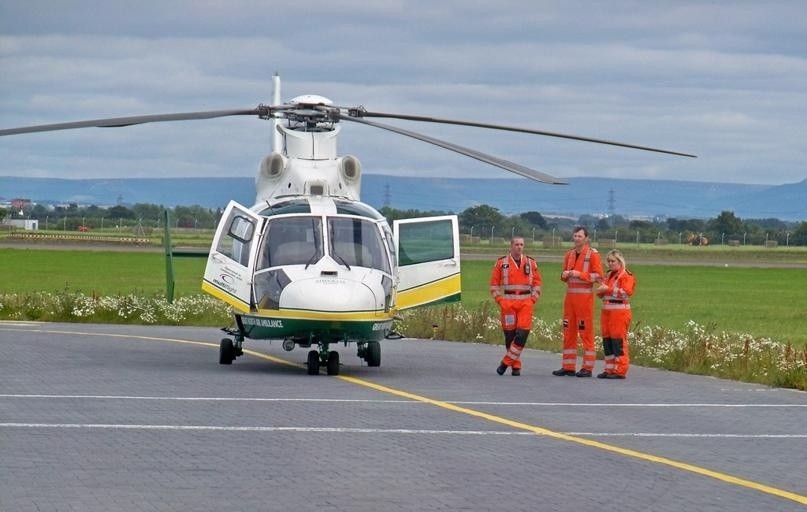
[552,227,603,377]
[594,247,634,378]
[488,236,542,377]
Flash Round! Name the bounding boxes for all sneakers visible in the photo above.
[552,368,625,379]
[497,361,507,375]
[512,369,520,376]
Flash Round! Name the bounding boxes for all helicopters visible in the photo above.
[0,70,698,375]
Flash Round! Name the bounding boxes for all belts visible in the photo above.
[608,299,623,304]
[505,290,530,294]
